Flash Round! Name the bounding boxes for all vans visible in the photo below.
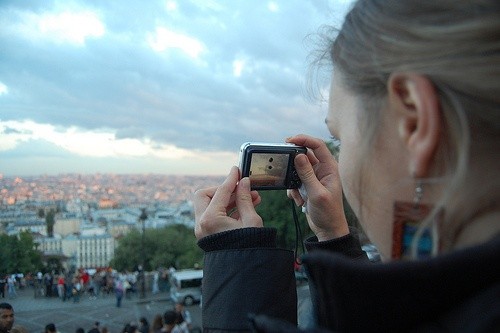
[170,269,203,305]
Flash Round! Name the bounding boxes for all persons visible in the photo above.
[190,1,499,333]
[0,266,203,333]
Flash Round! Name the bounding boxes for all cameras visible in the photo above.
[238,141,307,190]
